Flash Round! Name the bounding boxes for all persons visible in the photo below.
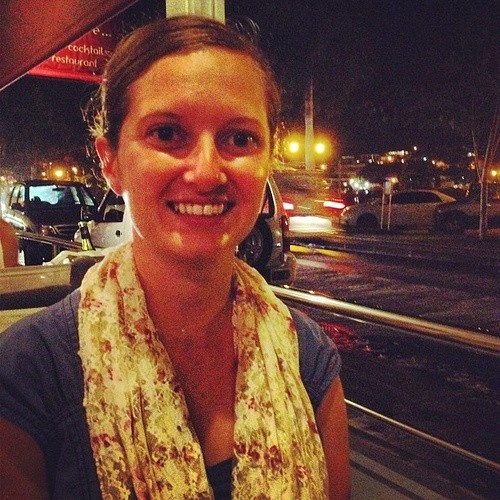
[0,14,355,499]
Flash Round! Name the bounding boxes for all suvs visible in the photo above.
[10,180,99,267]
[75,175,298,289]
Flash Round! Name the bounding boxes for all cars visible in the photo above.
[278,166,499,234]
